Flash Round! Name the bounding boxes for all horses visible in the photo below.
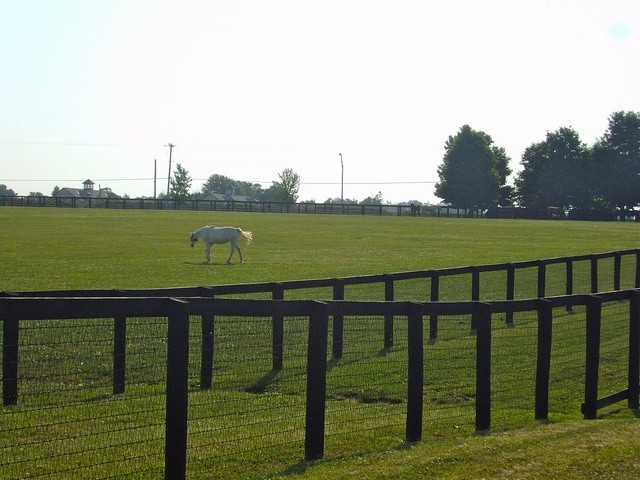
[189,225,254,264]
